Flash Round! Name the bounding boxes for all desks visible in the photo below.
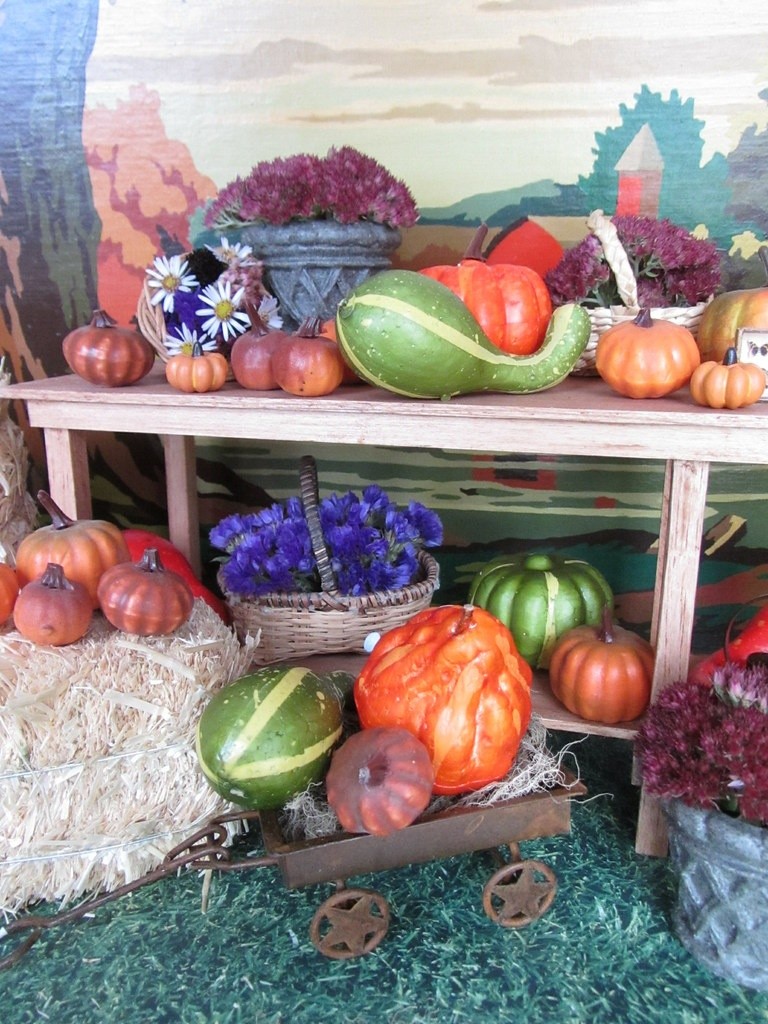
[4,351,767,857]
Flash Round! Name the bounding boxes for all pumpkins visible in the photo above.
[327,725,436,834]
[62,309,155,388]
[1,486,194,646]
[594,287,768,410]
[165,340,229,394]
[353,551,654,797]
[195,667,354,812]
[230,225,592,397]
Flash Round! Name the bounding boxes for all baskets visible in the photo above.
[551,207,715,379]
[217,454,439,667]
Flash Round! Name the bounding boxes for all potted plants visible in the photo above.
[194,145,419,337]
[619,652,768,994]
